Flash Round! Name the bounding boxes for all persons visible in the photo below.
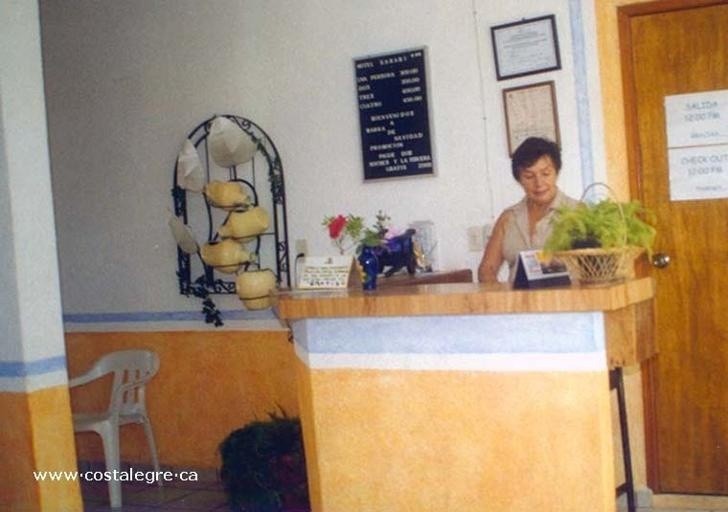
[478,137,586,282]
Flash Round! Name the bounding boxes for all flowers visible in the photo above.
[322,209,390,283]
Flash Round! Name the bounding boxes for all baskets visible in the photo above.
[554,182,644,282]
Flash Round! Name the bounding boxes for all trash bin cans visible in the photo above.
[219,417,310,512]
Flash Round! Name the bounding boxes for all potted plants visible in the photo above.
[537,199,656,283]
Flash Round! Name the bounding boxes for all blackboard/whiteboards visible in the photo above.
[351,45,439,183]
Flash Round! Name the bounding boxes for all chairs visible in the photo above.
[66,348,164,509]
